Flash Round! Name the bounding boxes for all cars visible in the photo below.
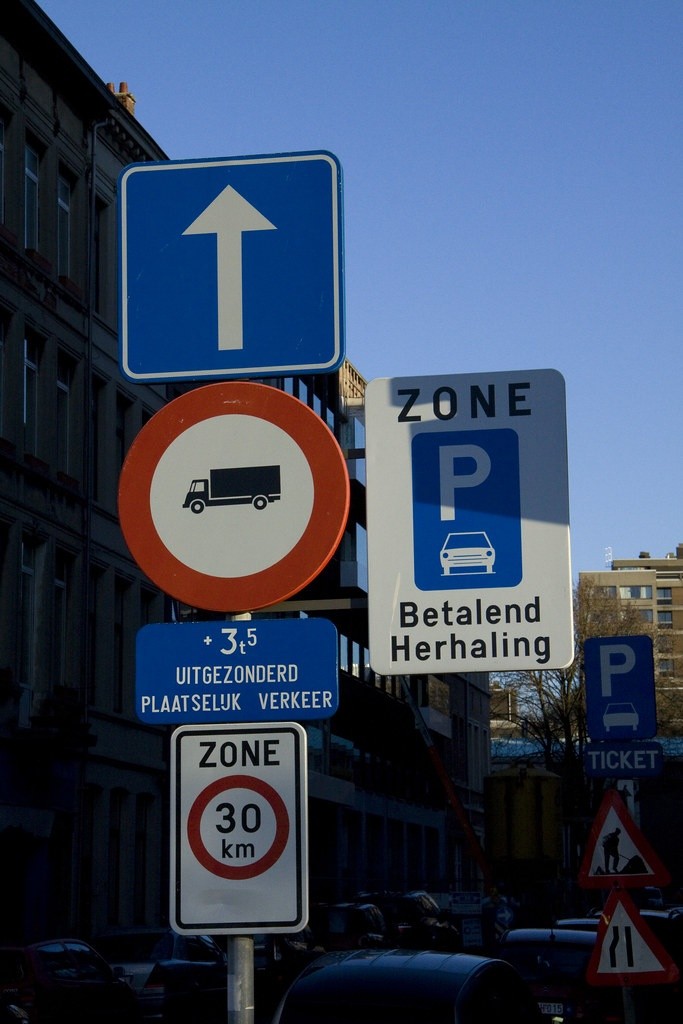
[95,928,227,1024]
[254,890,465,991]
[0,937,138,1024]
[271,948,541,1024]
[499,908,683,1023]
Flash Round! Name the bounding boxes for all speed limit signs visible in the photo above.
[170,723,308,934]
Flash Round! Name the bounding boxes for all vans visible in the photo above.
[644,887,664,909]
